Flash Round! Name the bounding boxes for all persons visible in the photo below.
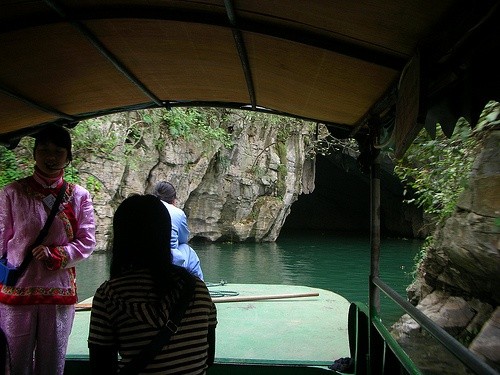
[86,195,218,374]
[0,123,98,375]
[152,180,204,282]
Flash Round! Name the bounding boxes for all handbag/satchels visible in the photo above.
[0,257,20,287]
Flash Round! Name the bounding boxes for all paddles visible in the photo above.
[74,290,318,309]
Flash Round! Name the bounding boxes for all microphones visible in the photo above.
[47,152,72,170]
